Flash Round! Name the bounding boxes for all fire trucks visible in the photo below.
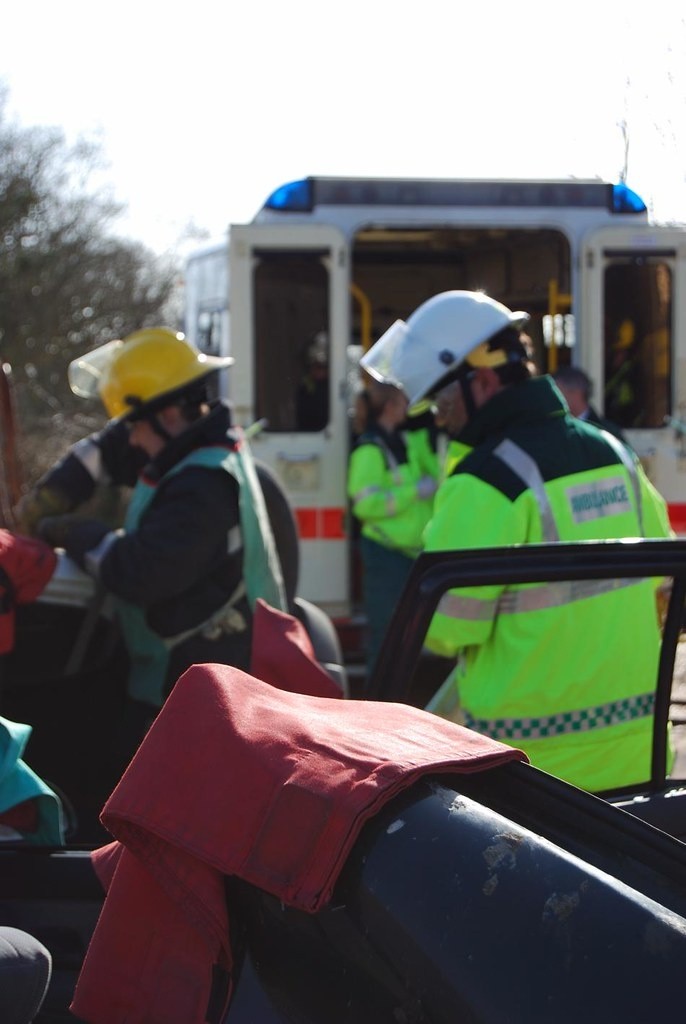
[182,175,686,681]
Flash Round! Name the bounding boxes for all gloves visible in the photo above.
[65,518,111,571]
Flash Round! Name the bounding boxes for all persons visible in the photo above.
[6,293,686,848]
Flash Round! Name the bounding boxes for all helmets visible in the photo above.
[359,290,529,416]
[68,327,235,437]
